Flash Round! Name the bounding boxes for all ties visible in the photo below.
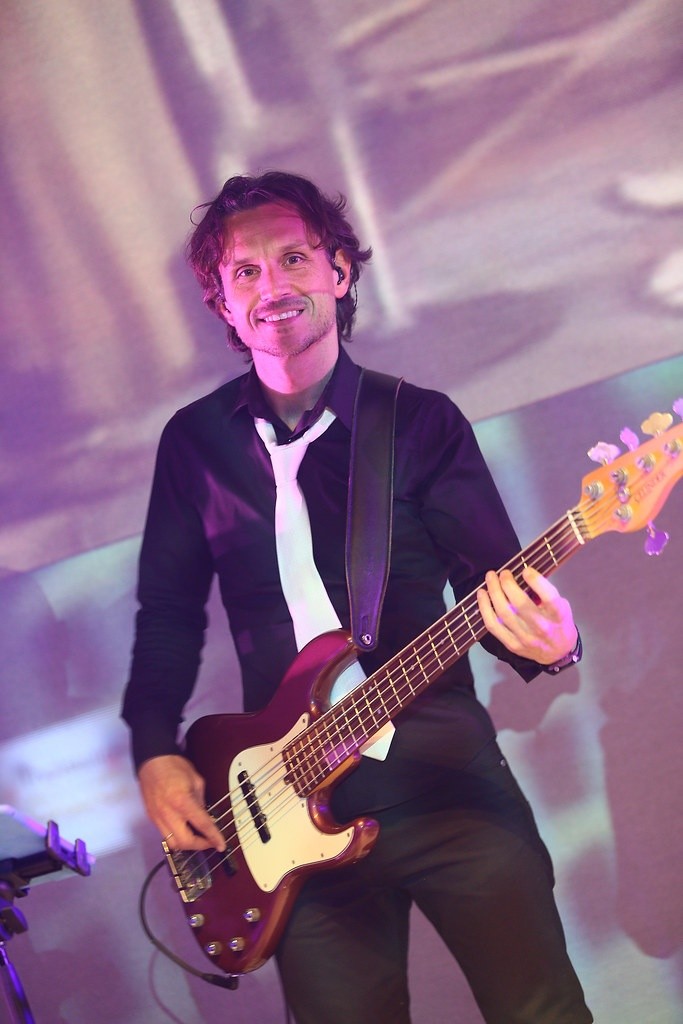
[250,412,397,762]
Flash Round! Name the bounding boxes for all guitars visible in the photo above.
[158,388,682,980]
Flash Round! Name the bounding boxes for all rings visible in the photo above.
[161,832,173,841]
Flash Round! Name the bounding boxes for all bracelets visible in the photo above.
[545,647,580,672]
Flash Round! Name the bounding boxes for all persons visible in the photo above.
[122,169,595,1024]
